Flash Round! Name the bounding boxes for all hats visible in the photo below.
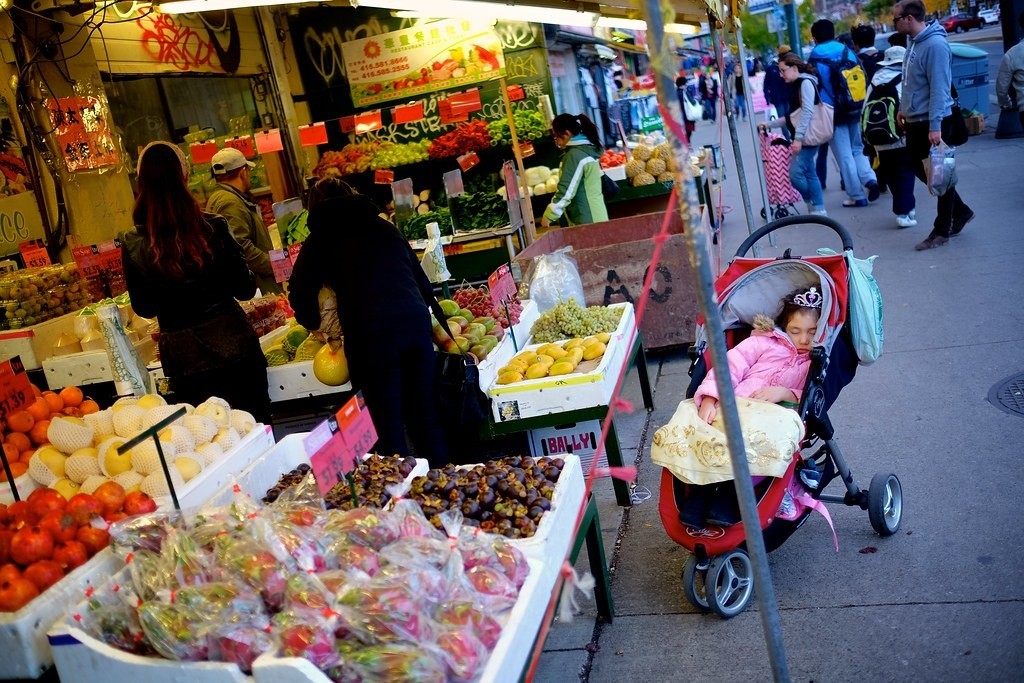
[779,45,791,54]
[875,46,906,66]
[211,147,256,174]
[136,139,191,179]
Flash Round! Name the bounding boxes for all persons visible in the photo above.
[542,112,610,227]
[677,281,824,526]
[758,53,828,217]
[847,22,886,80]
[289,177,454,467]
[807,17,881,208]
[892,1,977,252]
[835,32,856,50]
[994,25,1023,127]
[118,140,275,427]
[696,55,773,124]
[861,45,919,228]
[764,44,794,141]
[811,144,847,190]
[676,76,697,142]
[205,145,285,293]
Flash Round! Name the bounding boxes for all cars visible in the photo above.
[938,13,986,34]
[979,10,999,25]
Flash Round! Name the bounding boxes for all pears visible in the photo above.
[24,384,255,503]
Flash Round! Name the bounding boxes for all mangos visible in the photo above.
[497,332,608,389]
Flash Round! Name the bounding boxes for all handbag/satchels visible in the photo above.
[789,79,835,146]
[921,138,959,198]
[816,246,884,368]
[683,91,703,122]
[433,350,490,415]
[764,104,781,135]
[941,106,968,147]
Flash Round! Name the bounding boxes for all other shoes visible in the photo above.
[896,215,918,228]
[678,498,704,530]
[950,210,976,237]
[915,232,949,251]
[705,503,736,527]
[909,208,915,220]
[806,200,827,217]
[842,198,868,207]
[868,182,880,202]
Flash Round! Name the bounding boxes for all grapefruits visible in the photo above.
[312,341,351,387]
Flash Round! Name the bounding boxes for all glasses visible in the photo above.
[778,66,792,74]
[893,15,907,23]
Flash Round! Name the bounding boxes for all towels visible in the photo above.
[650,397,805,488]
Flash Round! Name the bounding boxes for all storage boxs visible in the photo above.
[2,270,661,683]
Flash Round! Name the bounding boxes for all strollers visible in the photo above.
[654,215,903,621]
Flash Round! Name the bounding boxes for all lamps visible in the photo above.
[351,0,602,27]
[597,14,700,37]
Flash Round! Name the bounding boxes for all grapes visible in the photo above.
[450,274,525,328]
[525,287,620,341]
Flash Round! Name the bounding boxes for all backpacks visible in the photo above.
[857,50,885,80]
[860,73,903,145]
[810,43,869,113]
